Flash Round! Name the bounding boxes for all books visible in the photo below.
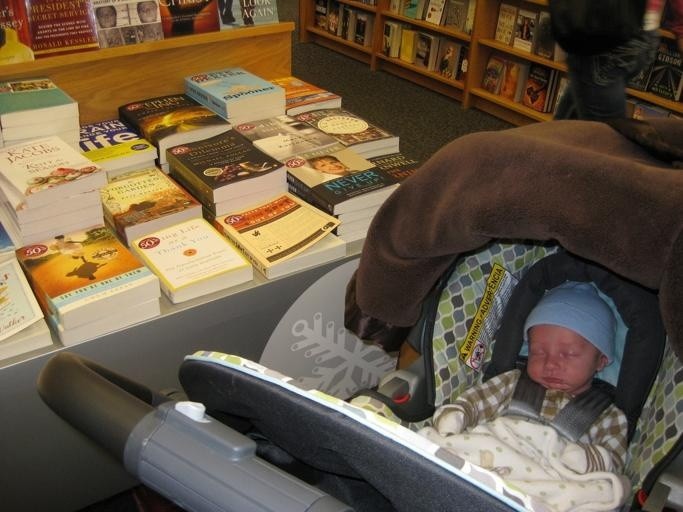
[1,1,280,63]
[483,2,568,115]
[315,1,475,81]
[1,66,429,360]
[625,44,683,119]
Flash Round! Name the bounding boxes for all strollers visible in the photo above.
[39,120,682,511]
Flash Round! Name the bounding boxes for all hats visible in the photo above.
[524,281,616,365]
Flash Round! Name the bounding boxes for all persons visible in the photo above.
[537,2,668,144]
[433,281,631,474]
